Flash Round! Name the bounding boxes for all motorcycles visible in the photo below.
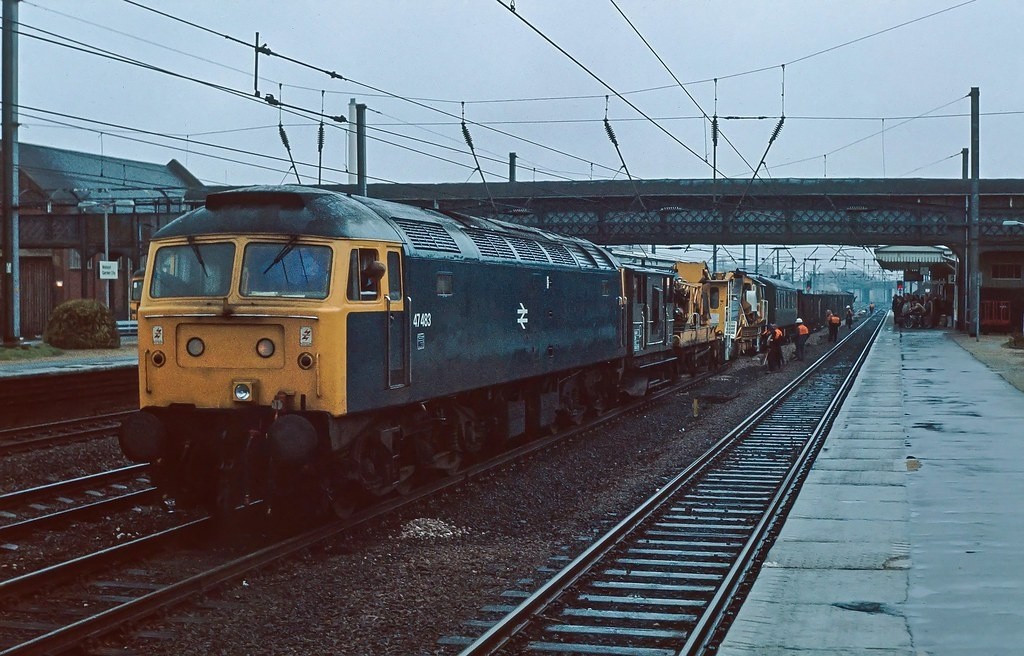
[904,308,933,329]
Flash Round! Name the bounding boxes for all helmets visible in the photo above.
[846,305,850,308]
[795,318,803,323]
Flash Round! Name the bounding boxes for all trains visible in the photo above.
[116,187,857,535]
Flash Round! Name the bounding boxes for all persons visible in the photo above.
[792,318,810,361]
[761,323,786,374]
[826,310,841,344]
[865,293,946,329]
[348,260,385,300]
[845,305,853,329]
